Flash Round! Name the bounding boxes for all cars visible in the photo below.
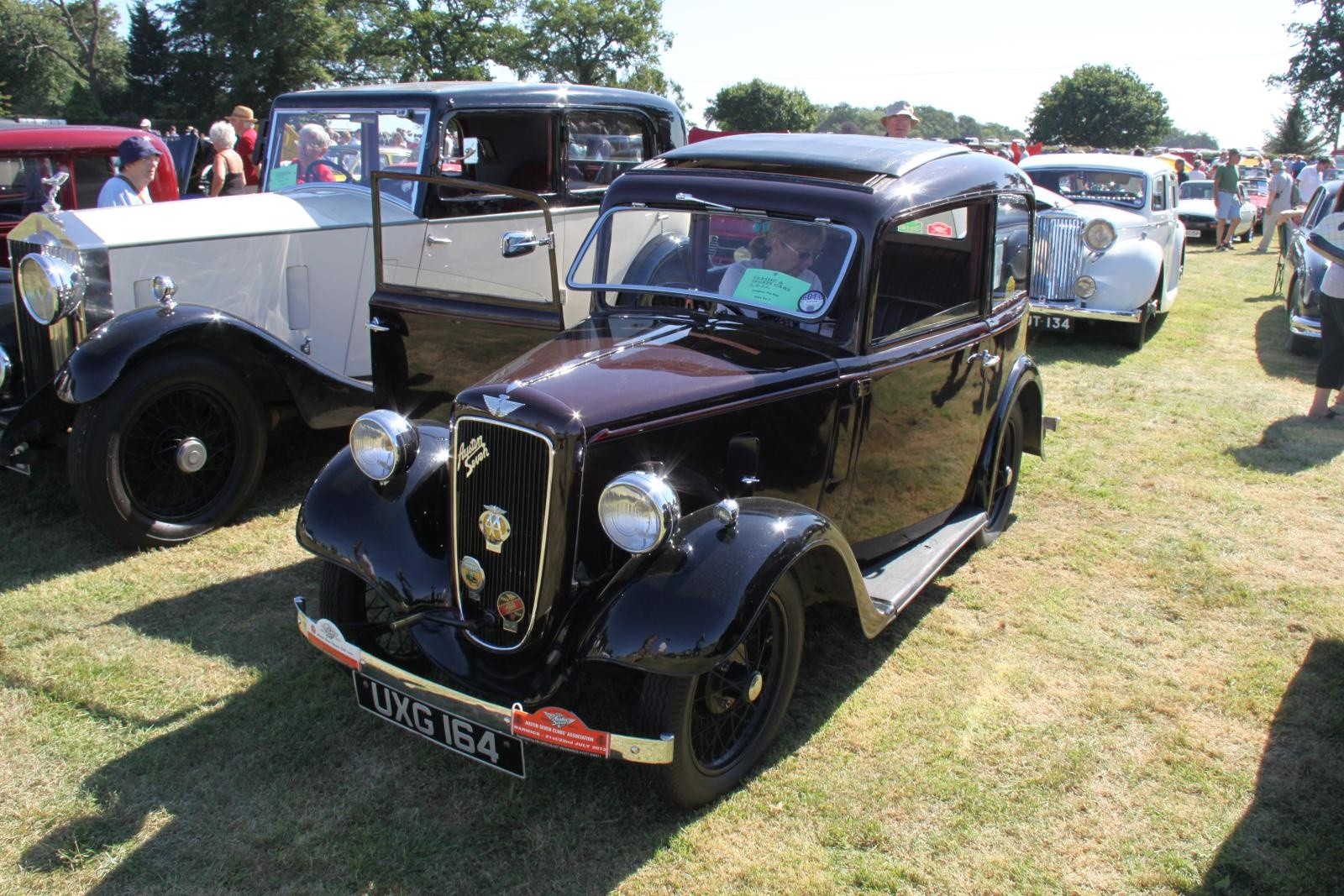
[912,134,1344,243]
[0,108,774,268]
[0,75,689,541]
[949,153,1188,352]
[289,132,1064,811]
[1284,177,1344,359]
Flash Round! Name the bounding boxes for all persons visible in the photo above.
[1296,159,1331,206]
[1130,145,1320,188]
[224,105,262,185]
[140,119,213,144]
[567,119,636,184]
[207,121,247,196]
[1251,159,1293,251]
[1309,187,1344,418]
[714,220,827,335]
[289,123,335,184]
[1214,149,1241,251]
[325,128,457,174]
[1042,145,1117,154]
[948,137,1029,165]
[97,136,163,207]
[880,100,919,137]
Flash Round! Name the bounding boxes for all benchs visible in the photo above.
[481,157,640,191]
[1074,189,1139,204]
[758,233,970,326]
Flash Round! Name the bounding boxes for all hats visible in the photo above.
[1272,159,1287,169]
[880,100,919,129]
[224,105,258,123]
[140,119,151,127]
[118,135,163,171]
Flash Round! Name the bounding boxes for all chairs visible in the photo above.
[1273,222,1287,295]
[1192,189,1204,199]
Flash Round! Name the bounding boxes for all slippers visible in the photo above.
[1306,410,1344,420]
[1330,402,1344,414]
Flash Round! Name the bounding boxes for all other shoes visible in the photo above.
[1223,241,1236,250]
[1215,245,1228,251]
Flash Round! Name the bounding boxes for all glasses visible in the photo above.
[137,156,160,164]
[294,139,320,148]
[779,239,820,262]
